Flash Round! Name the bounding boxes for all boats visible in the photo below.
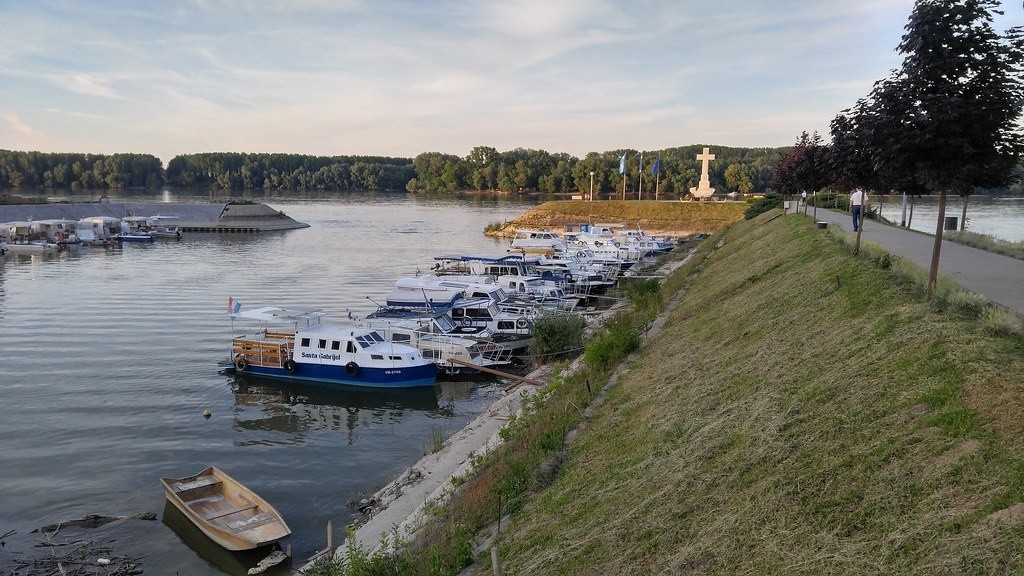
[218,297,439,386]
[347,223,676,376]
[0,215,183,251]
[160,466,292,551]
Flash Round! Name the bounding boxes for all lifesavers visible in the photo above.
[284,359,297,373]
[674,235,679,240]
[236,358,248,371]
[345,362,359,376]
[580,266,586,270]
[60,232,64,243]
[615,242,620,248]
[565,273,572,280]
[652,236,655,240]
[578,241,584,245]
[516,317,528,328]
[461,315,473,327]
[577,252,586,257]
[587,260,594,266]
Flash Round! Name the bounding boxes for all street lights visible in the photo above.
[589,172,594,201]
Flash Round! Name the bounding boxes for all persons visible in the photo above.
[802,190,806,206]
[57,231,64,242]
[850,186,869,232]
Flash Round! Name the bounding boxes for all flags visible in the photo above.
[651,158,659,174]
[225,297,241,321]
[618,154,626,173]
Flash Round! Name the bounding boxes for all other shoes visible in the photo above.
[853,229,857,231]
